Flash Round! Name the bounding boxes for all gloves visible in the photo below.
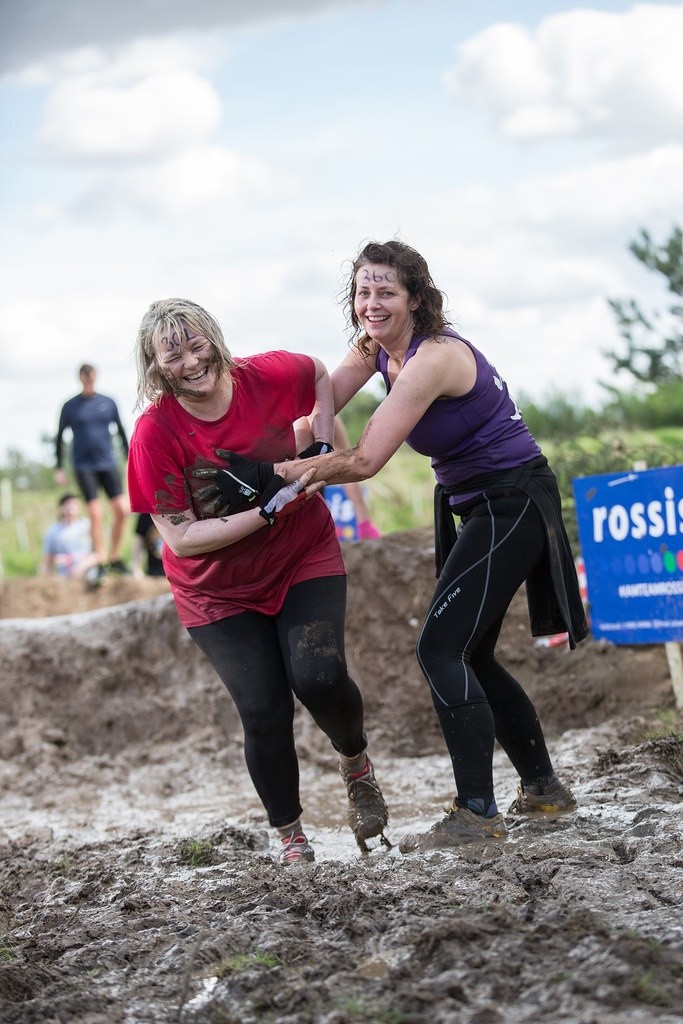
[193,448,274,518]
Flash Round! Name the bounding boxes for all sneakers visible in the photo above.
[399,797,509,853]
[276,831,316,868]
[507,779,580,816]
[339,750,388,839]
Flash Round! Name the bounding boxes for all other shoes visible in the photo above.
[108,560,133,574]
[86,560,104,590]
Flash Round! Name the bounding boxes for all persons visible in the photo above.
[44,363,380,582]
[190,240,589,855]
[125,298,390,866]
